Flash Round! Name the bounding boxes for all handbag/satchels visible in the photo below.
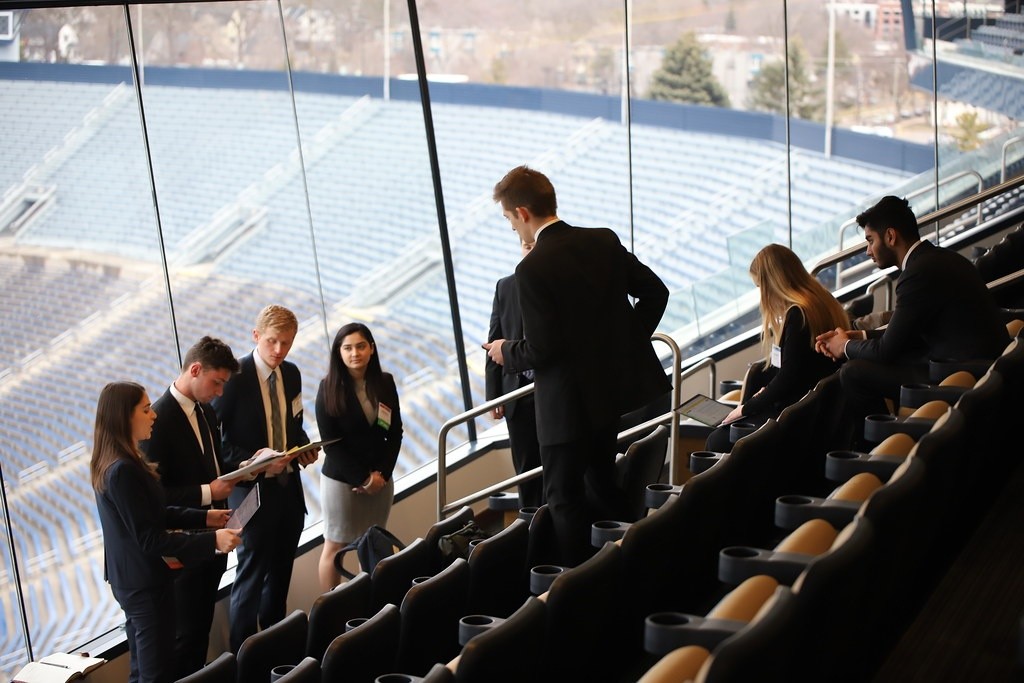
[438,520,489,565]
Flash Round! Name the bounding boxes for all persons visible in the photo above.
[316,323,403,593]
[812,196,1012,415]
[134,336,241,680]
[89,382,243,683]
[487,166,658,568]
[207,305,321,658]
[704,243,850,454]
[484,233,544,511]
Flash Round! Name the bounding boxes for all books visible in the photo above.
[217,451,286,481]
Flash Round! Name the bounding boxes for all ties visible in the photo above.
[523,335,535,379]
[194,403,224,509]
[269,371,288,489]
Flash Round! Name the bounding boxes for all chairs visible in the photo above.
[0,137,1024,683]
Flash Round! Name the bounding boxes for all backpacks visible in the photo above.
[333,524,407,579]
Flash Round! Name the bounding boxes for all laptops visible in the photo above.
[673,393,748,428]
[161,483,261,569]
